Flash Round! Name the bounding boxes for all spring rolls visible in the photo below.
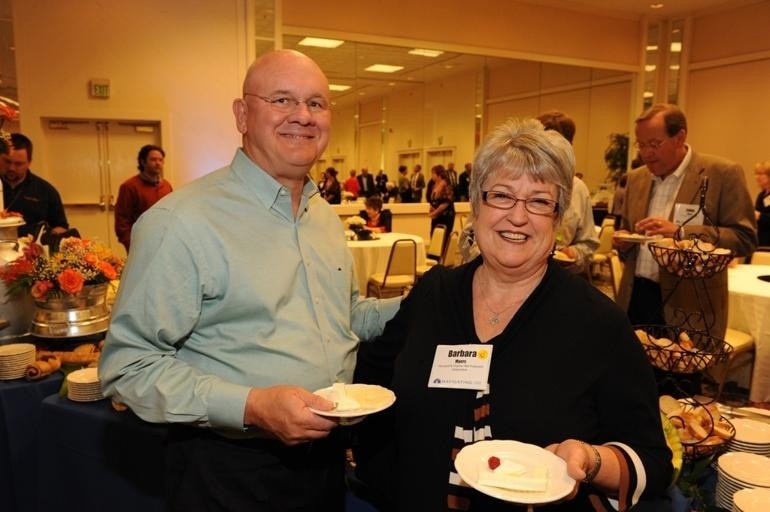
[25,339,107,380]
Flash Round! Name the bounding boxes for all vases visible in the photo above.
[28,284,113,355]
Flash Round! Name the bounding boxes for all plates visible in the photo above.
[310,384,406,420]
[0,342,36,381]
[614,234,669,246]
[455,437,574,503]
[66,364,106,402]
[715,403,770,511]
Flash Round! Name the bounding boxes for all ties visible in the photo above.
[450,172,454,185]
[413,176,416,186]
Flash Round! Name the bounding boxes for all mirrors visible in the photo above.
[275,21,641,213]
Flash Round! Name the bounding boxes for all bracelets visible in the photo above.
[578,441,605,491]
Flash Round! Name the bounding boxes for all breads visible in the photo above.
[654,234,730,276]
[659,395,734,453]
[635,330,712,374]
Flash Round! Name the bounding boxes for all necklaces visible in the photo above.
[478,264,546,331]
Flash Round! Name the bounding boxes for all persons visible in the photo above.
[352,116,676,511]
[575,158,644,230]
[531,111,598,276]
[112,142,177,256]
[1,132,71,238]
[750,160,770,250]
[98,47,406,508]
[616,106,757,398]
[312,161,473,265]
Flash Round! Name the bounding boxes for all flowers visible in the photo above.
[0,100,23,159]
[0,234,126,308]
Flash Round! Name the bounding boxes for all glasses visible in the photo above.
[480,190,559,216]
[633,136,667,149]
[243,93,328,112]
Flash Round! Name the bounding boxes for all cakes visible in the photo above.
[479,450,548,493]
[0,207,26,225]
[328,381,394,412]
[0,235,34,267]
